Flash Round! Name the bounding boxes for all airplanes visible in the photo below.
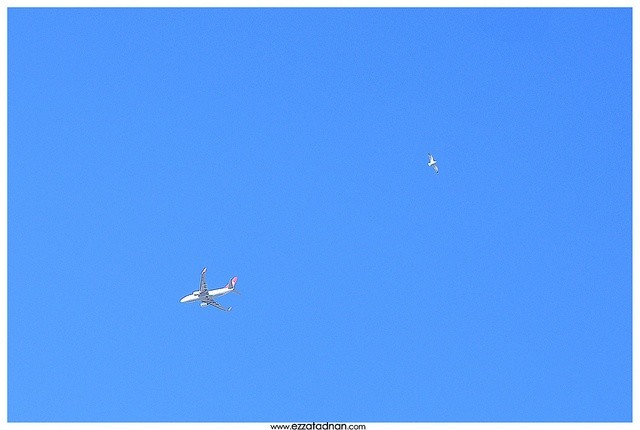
[179,267,240,312]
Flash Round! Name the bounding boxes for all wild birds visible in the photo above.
[427,153,439,174]
